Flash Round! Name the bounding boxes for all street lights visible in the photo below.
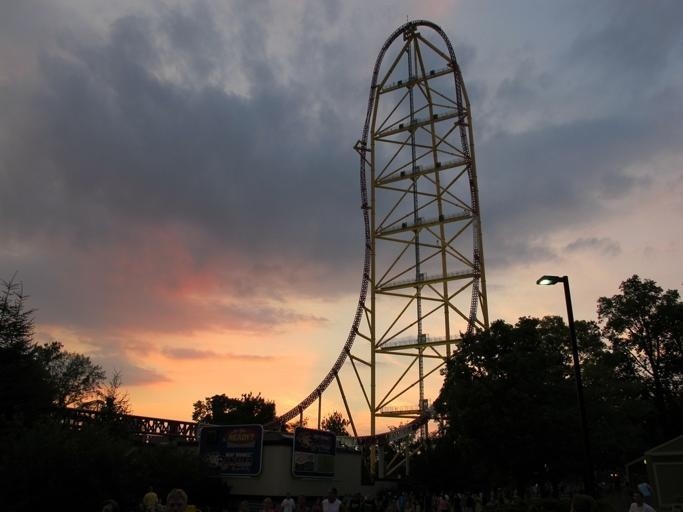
[535,275,599,496]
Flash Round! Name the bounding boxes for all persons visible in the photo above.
[238,476,658,512]
[101,484,196,512]
